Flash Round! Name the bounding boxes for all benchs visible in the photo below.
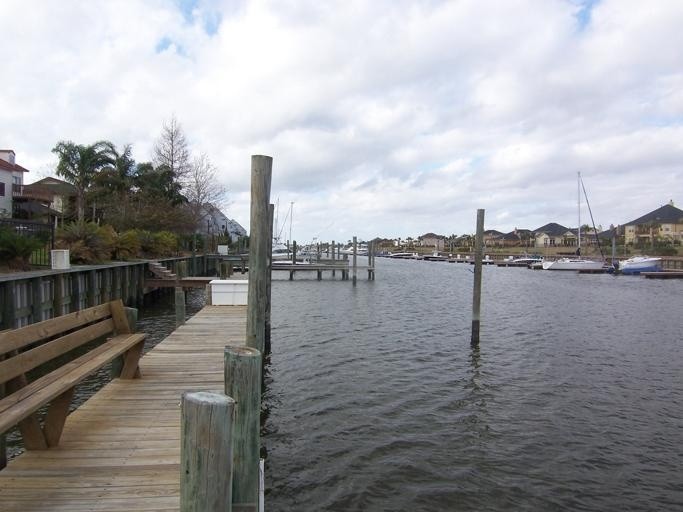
[0,298,147,463]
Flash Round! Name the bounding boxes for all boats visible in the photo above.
[618,256,662,275]
[542,258,615,270]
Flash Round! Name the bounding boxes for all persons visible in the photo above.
[576,248,581,256]
